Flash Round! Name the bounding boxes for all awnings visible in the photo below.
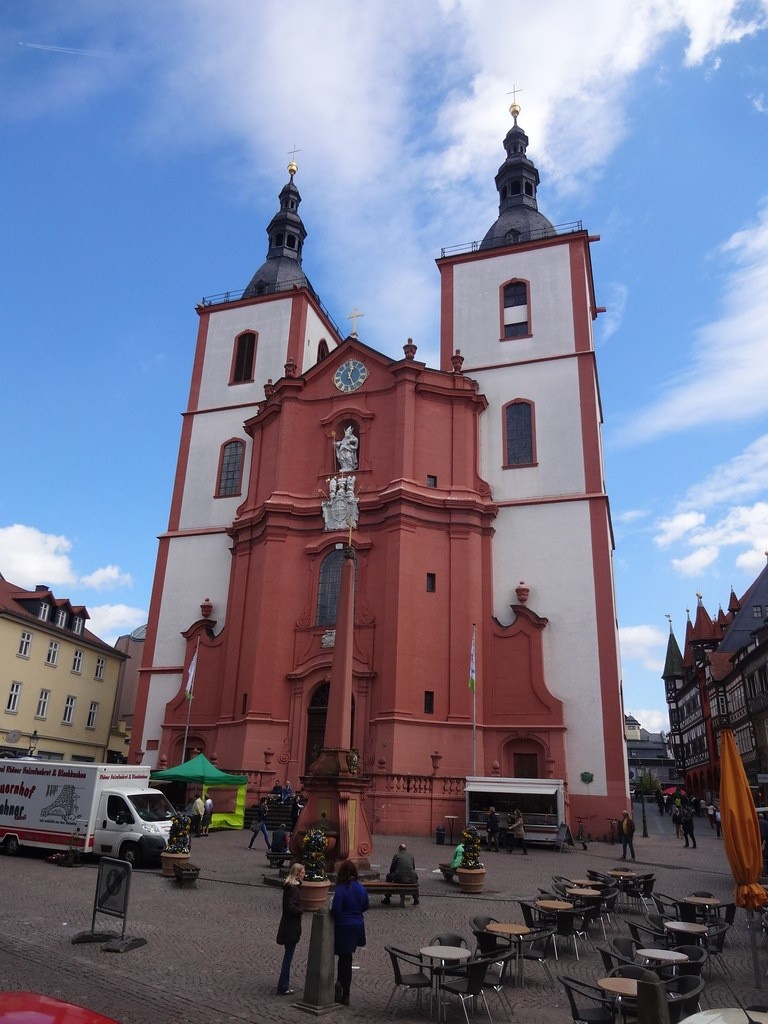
[464,786,557,794]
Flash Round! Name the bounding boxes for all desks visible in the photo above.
[635,948,688,975]
[570,879,603,885]
[664,922,709,933]
[597,977,639,1024]
[567,888,601,895]
[606,871,636,882]
[419,945,471,1022]
[535,900,574,909]
[486,923,530,981]
[682,896,721,906]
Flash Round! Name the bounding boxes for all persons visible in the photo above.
[380,843,419,905]
[705,802,722,837]
[334,426,358,470]
[275,863,306,995]
[486,806,528,855]
[290,795,303,835]
[331,861,369,1006]
[619,810,635,861]
[271,824,289,867]
[247,797,271,850]
[185,793,213,838]
[682,808,697,849]
[654,787,705,817]
[758,814,768,841]
[271,780,292,805]
[671,808,684,839]
[450,839,484,868]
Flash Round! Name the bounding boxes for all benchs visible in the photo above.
[361,880,420,908]
[266,852,293,867]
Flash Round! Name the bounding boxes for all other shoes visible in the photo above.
[335,983,350,1006]
[506,848,513,854]
[618,856,626,859]
[683,845,689,847]
[247,847,257,850]
[201,833,209,837]
[521,850,528,855]
[492,847,500,852]
[630,857,636,861]
[381,898,391,905]
[414,899,420,905]
[485,847,492,852]
[278,989,295,994]
[691,845,696,849]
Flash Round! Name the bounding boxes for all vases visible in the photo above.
[301,878,331,910]
[162,852,191,875]
[457,869,487,893]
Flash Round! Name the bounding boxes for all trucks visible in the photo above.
[0,756,192,870]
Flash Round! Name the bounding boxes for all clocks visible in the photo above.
[333,360,369,394]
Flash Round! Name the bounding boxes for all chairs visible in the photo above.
[385,867,768,1024]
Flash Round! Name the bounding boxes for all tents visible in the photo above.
[150,752,248,830]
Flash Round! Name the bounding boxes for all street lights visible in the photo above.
[638,770,649,838]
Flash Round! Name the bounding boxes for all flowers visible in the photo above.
[459,822,484,870]
[302,827,327,882]
[165,809,191,854]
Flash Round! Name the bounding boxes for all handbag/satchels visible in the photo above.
[250,820,262,833]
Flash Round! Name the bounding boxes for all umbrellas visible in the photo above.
[719,727,768,989]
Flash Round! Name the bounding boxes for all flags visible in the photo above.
[185,653,196,699]
[468,640,475,689]
